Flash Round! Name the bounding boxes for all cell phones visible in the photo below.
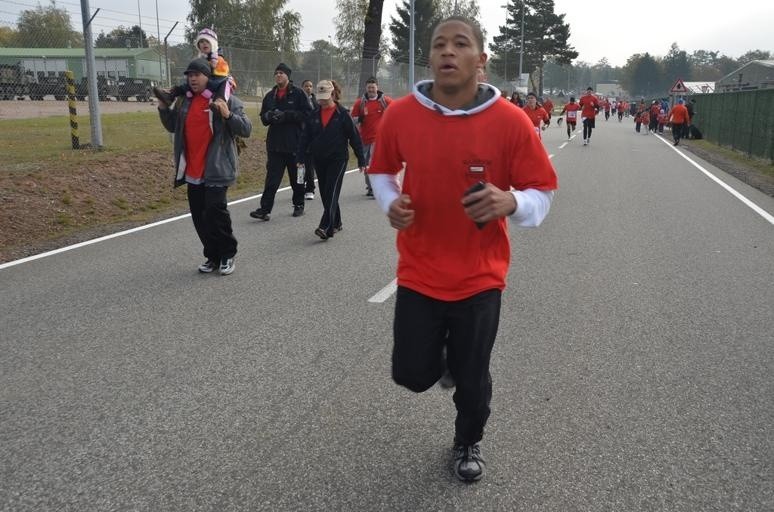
[464,182,485,229]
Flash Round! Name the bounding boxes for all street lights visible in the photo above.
[327,35,333,82]
[163,20,181,87]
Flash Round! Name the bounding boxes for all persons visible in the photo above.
[543,96,554,119]
[523,92,550,140]
[579,87,599,145]
[248,61,305,222]
[602,99,636,122]
[155,58,252,275]
[303,155,316,199]
[510,91,523,108]
[559,97,581,139]
[299,80,366,238]
[351,77,394,199]
[151,28,236,113]
[363,14,558,486]
[303,79,320,110]
[634,97,697,146]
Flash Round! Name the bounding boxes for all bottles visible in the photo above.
[297,164,304,184]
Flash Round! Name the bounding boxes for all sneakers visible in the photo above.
[198,259,220,273]
[305,192,314,199]
[209,102,223,121]
[292,201,304,217]
[315,227,329,239]
[366,188,374,196]
[588,138,591,144]
[584,139,588,145]
[250,208,271,221]
[152,85,174,107]
[218,257,236,276]
[438,336,456,389]
[451,438,486,482]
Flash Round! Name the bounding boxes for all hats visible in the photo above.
[195,28,219,69]
[275,63,292,80]
[184,58,211,79]
[316,80,335,101]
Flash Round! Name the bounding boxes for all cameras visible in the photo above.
[272,107,281,121]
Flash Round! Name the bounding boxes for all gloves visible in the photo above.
[273,110,284,120]
[358,115,364,122]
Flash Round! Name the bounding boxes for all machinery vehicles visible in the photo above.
[29,69,66,102]
[113,76,158,103]
[0,65,34,101]
[76,72,118,103]
